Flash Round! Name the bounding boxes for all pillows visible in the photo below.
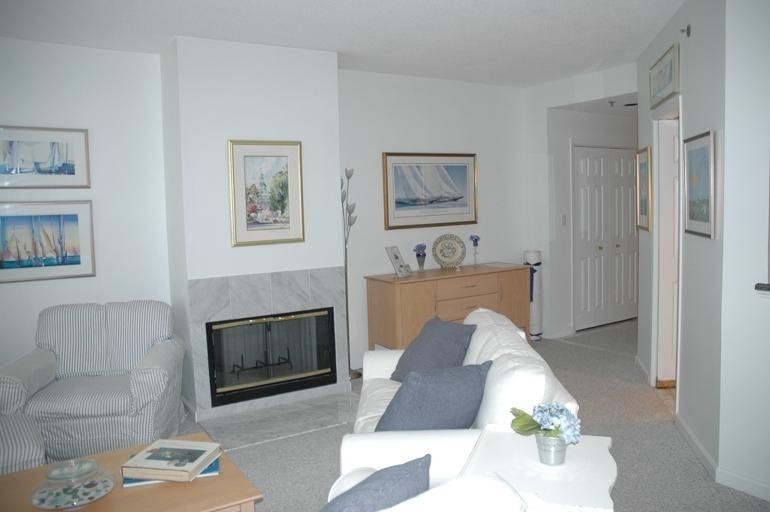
[375,308,555,431]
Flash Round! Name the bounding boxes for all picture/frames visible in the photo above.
[385,245,410,278]
[0,200,97,283]
[635,146,652,234]
[228,139,305,247]
[647,39,680,110]
[683,129,716,240]
[0,124,91,189]
[383,152,479,230]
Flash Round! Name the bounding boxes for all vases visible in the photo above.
[536,434,566,466]
[416,251,425,273]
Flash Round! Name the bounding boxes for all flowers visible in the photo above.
[414,244,426,253]
[510,399,583,445]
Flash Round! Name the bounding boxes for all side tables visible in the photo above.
[464,423,618,512]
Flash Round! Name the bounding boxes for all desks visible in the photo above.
[366,262,531,350]
[0,432,263,512]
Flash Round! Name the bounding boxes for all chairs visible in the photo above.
[0,300,187,464]
[328,464,537,512]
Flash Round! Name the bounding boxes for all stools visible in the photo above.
[0,414,48,474]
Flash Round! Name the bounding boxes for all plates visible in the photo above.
[433,234,466,267]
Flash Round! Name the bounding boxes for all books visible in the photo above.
[122,454,219,488]
[121,438,223,483]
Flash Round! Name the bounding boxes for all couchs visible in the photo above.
[339,331,580,477]
